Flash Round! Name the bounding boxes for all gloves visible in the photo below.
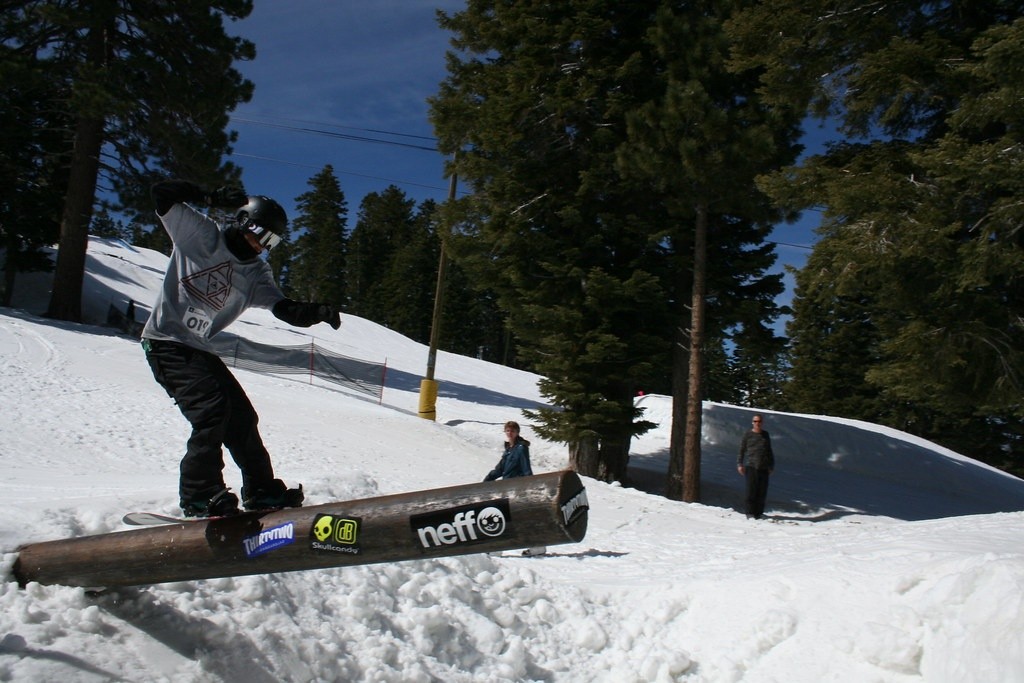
[198,185,249,209]
[305,301,341,330]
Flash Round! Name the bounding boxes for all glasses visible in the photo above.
[753,420,761,423]
[258,230,282,251]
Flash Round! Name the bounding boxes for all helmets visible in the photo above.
[234,195,287,236]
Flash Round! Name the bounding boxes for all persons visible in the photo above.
[142,172,341,516]
[737,414,774,519]
[483,422,546,557]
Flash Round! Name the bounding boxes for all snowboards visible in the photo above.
[122,497,302,526]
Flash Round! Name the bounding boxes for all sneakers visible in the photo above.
[243,479,304,510]
[182,493,238,517]
